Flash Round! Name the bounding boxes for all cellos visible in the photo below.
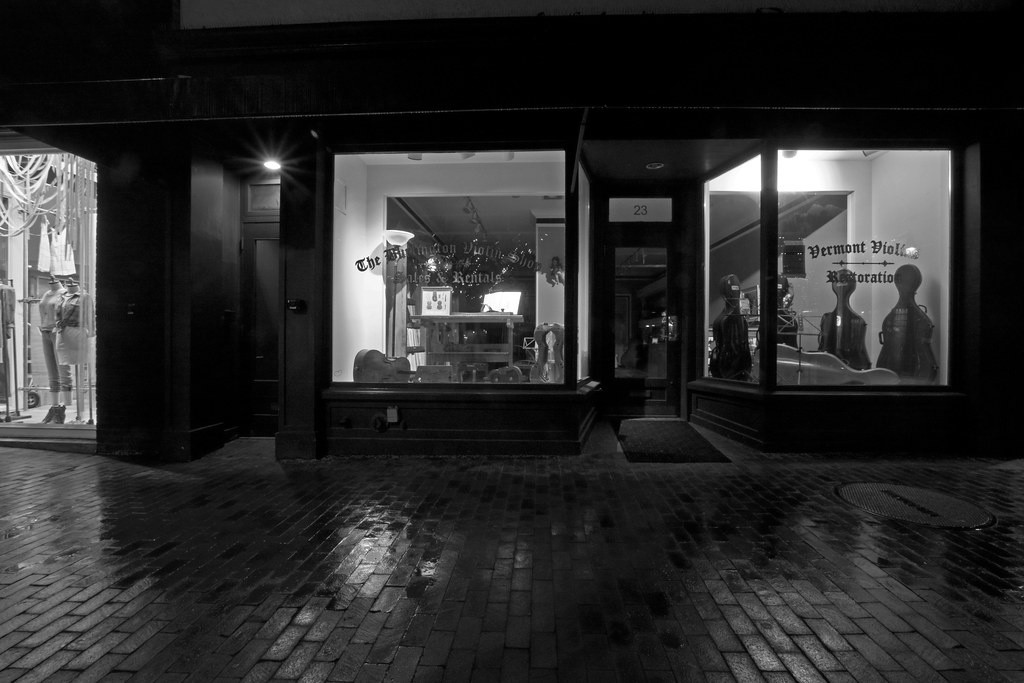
[354,348,424,382]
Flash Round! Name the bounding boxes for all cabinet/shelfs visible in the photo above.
[409,313,524,370]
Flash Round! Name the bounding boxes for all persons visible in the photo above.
[55,282,95,366]
[39,281,72,425]
[0,283,16,364]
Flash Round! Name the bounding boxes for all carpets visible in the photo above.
[611,420,732,463]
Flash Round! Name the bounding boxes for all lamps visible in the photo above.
[408,153,422,160]
[385,230,415,356]
[463,201,481,234]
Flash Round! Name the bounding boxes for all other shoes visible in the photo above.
[41,407,58,423]
[49,405,65,424]
